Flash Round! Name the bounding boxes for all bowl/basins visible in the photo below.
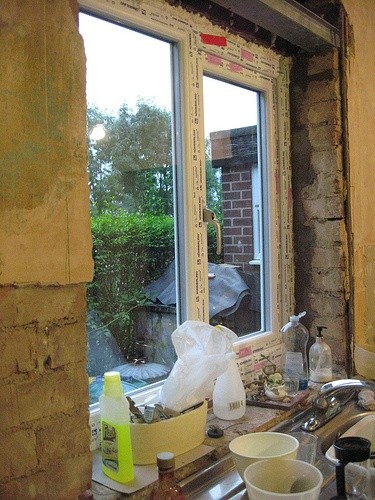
[130,400,209,465]
[229,432,299,482]
[243,459,324,500]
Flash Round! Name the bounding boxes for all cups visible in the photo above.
[289,432,317,466]
[282,373,299,397]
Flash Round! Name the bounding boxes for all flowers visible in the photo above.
[265,372,284,388]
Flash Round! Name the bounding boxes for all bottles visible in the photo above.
[149,452,185,500]
[99,371,134,483]
[279,310,309,390]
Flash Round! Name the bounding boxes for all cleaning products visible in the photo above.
[99,370,135,483]
[213,324,247,422]
[279,311,309,391]
[309,325,333,383]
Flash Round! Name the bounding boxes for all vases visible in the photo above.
[265,385,285,401]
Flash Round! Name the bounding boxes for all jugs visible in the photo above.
[319,436,375,500]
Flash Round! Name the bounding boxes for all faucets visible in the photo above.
[300,379,375,432]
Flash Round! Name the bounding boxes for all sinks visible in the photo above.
[315,412,375,475]
